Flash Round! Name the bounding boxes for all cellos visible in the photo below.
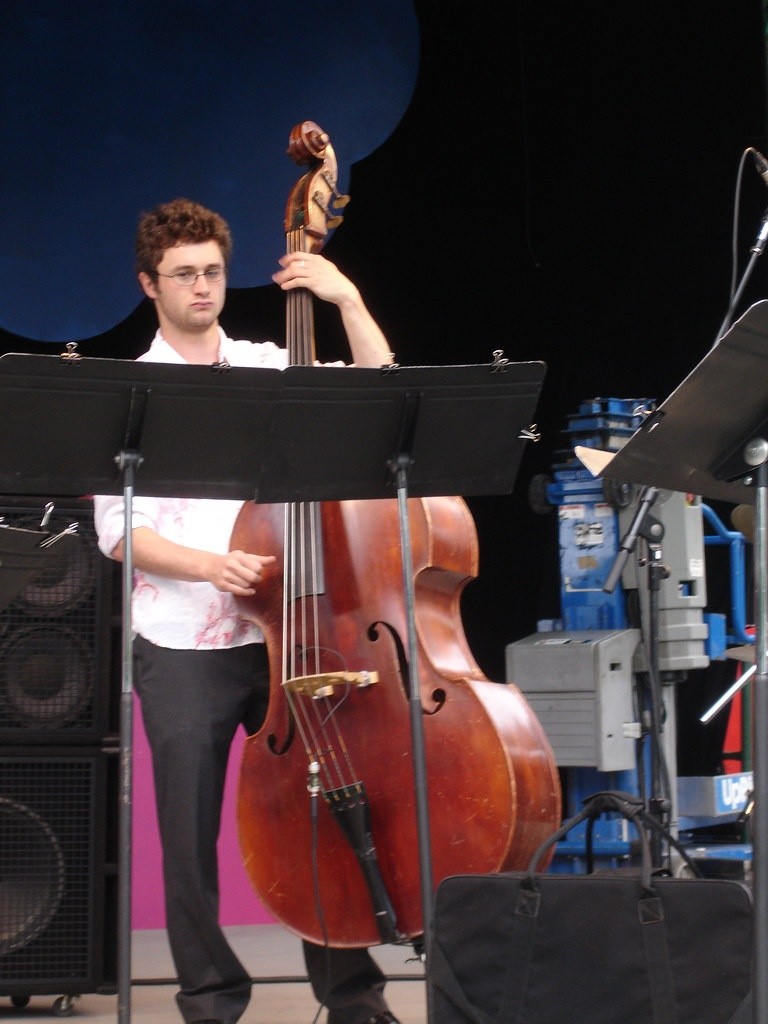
[232,121,566,949]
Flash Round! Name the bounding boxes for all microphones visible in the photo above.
[754,151,768,185]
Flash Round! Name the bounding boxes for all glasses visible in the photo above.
[151,267,230,286]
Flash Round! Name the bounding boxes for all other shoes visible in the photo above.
[327,1009,399,1024]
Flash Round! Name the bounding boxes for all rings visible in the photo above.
[300,261,303,267]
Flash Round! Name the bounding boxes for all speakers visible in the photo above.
[0,494,126,996]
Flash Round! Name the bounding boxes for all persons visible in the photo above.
[92,197,399,1024]
